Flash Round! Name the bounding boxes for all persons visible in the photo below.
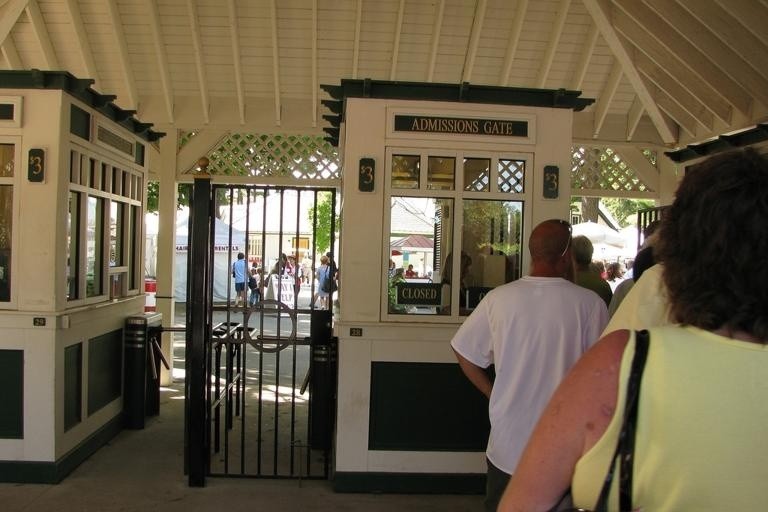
[494,141,768,512]
[439,249,472,315]
[388,259,433,279]
[231,251,338,314]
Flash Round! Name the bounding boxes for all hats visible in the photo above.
[288,255,296,259]
[326,252,331,256]
[572,236,593,255]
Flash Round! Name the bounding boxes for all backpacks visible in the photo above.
[249,278,257,289]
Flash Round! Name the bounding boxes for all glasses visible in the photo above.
[555,220,572,256]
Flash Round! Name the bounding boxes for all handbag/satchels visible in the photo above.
[233,271,235,277]
[322,279,338,293]
